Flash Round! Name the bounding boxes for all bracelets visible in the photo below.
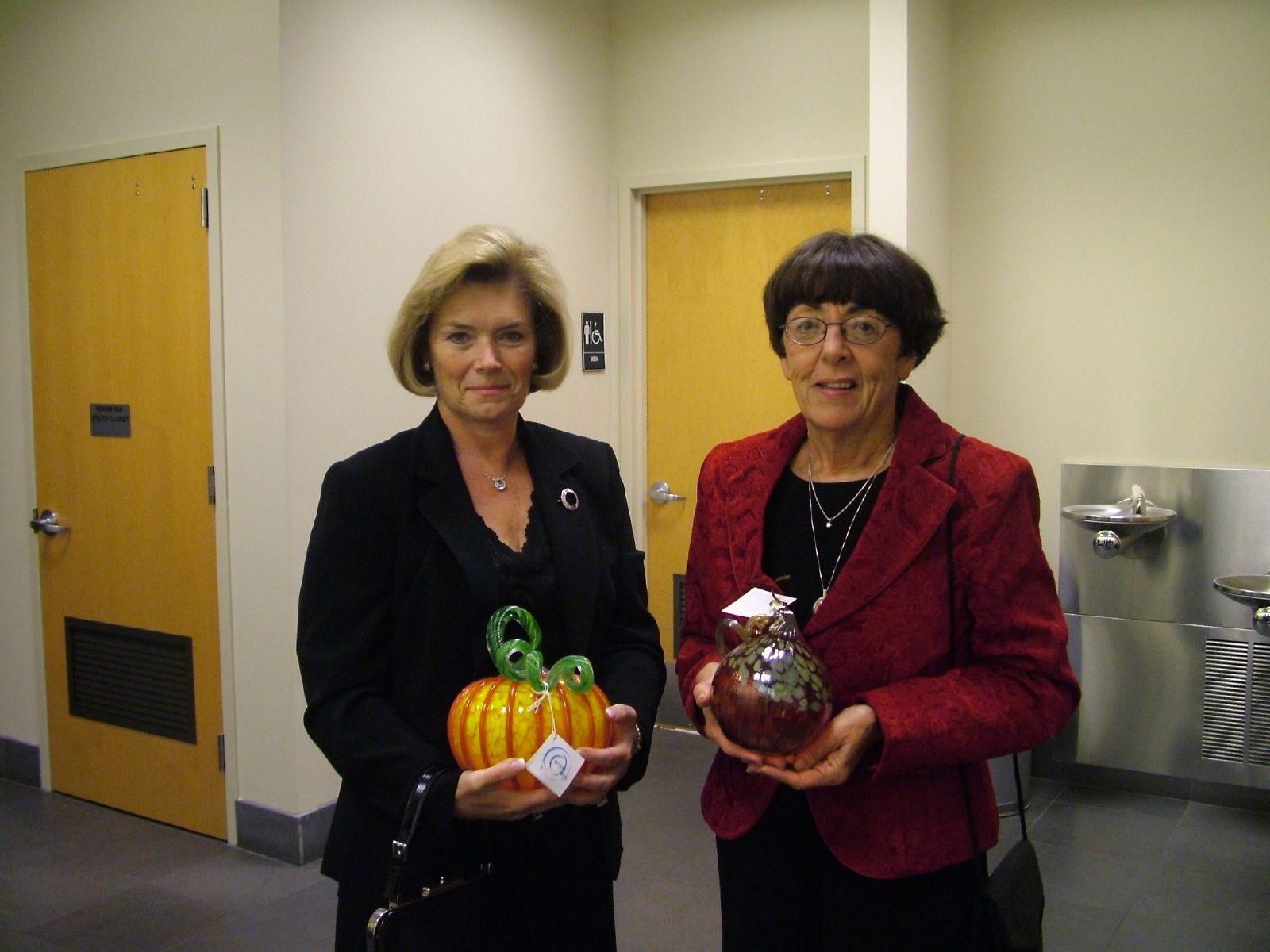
[635,723,641,753]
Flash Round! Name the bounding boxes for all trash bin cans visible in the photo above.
[985,751,1033,819]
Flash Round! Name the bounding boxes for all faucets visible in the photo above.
[1134,494,1148,515]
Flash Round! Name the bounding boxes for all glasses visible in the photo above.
[778,316,899,345]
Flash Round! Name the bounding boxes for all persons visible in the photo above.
[296,225,669,952]
[675,229,1081,952]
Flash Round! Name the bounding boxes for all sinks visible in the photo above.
[1061,502,1177,532]
[1214,574,1269,605]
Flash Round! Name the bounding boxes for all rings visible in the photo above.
[598,792,608,806]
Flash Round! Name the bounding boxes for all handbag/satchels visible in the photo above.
[978,839,1045,951]
[365,765,494,952]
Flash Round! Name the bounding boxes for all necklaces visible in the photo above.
[454,446,520,490]
[806,437,898,615]
